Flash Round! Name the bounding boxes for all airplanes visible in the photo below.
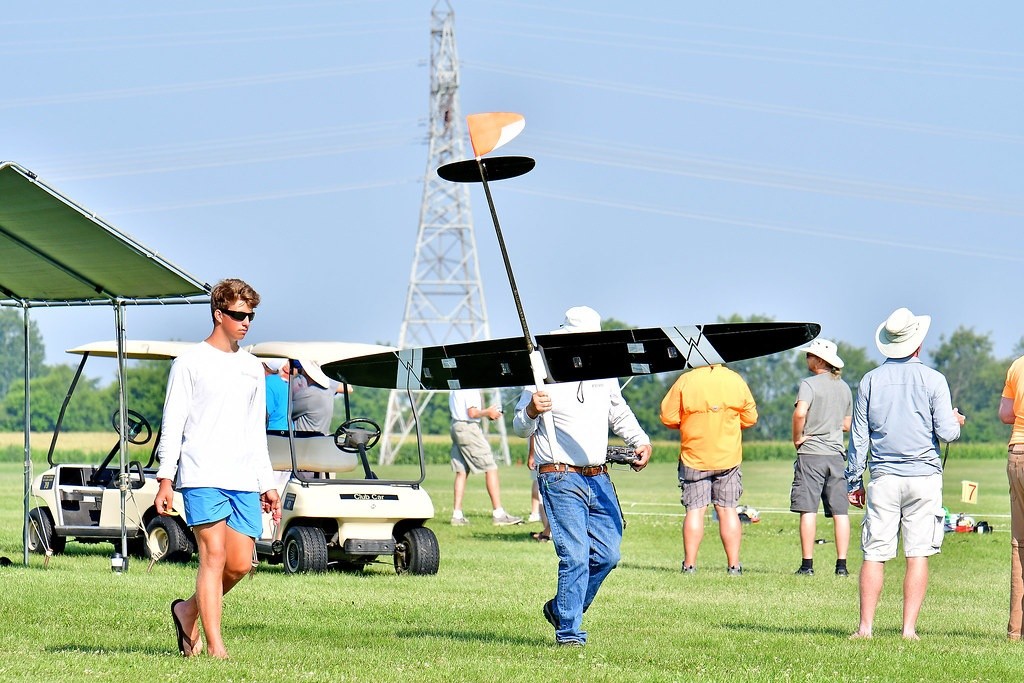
[320,112,821,472]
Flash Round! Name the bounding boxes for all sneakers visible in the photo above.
[451,517,469,526]
[492,514,521,527]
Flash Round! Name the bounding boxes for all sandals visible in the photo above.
[530,532,549,543]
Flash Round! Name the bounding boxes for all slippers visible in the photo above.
[171,599,201,658]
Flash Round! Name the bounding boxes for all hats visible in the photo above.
[800,338,844,368]
[876,308,931,359]
[299,359,329,388]
[548,307,602,333]
[262,357,281,371]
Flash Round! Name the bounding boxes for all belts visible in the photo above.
[538,463,608,477]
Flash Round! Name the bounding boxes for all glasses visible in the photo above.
[217,306,254,321]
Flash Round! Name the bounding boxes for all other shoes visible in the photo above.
[836,568,848,576]
[795,566,814,575]
[543,599,582,648]
[529,514,543,523]
[727,566,742,576]
[682,561,693,574]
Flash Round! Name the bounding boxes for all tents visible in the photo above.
[0,162,211,571]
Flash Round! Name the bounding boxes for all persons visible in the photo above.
[528,472,551,541]
[660,366,757,575]
[264,360,353,434]
[449,390,523,527]
[155,280,283,660]
[846,307,966,641]
[512,305,651,645]
[791,340,850,576]
[999,354,1024,637]
